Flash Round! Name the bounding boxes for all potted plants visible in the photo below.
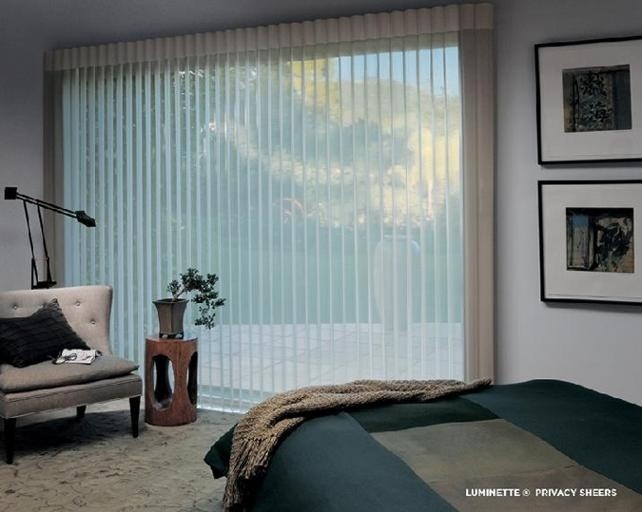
[152,269,228,337]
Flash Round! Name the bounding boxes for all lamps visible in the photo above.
[3,184,99,288]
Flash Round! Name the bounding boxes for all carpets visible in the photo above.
[0,408,251,512]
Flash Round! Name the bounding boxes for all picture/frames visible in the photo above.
[534,37,642,164]
[537,177,641,306]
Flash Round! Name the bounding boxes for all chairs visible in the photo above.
[0,285,143,466]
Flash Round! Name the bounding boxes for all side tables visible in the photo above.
[146,335,198,425]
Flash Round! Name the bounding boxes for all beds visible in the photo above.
[230,378,641,508]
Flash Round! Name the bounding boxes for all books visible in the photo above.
[62,348,100,365]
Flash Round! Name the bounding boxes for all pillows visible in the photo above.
[0,297,92,367]
